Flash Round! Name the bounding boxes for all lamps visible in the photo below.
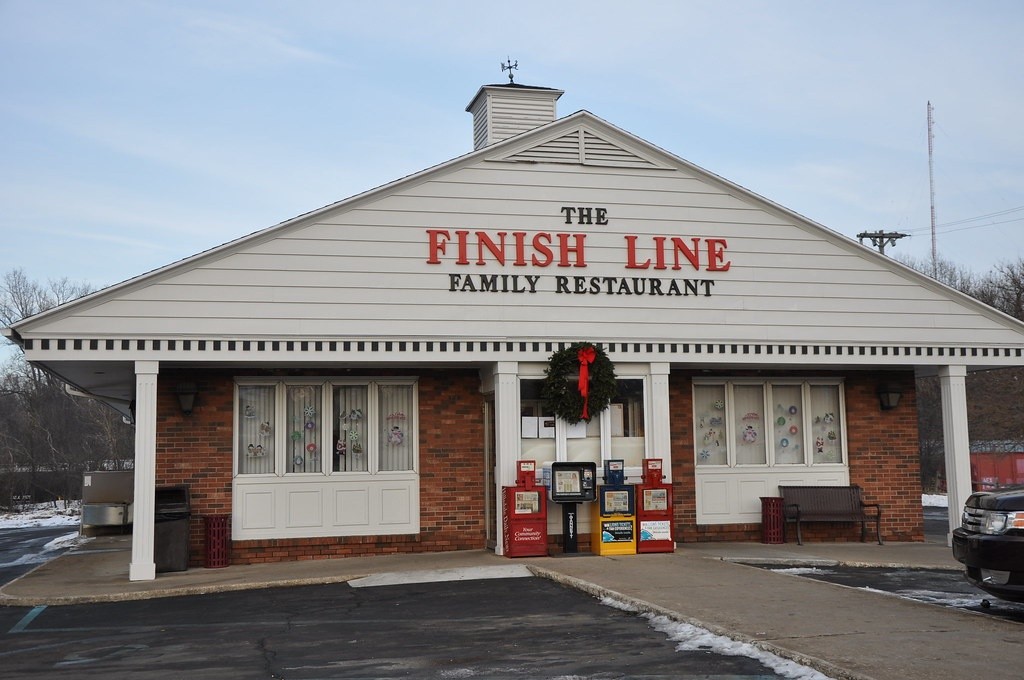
[877,376,903,409]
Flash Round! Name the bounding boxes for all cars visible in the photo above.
[951,484,1024,604]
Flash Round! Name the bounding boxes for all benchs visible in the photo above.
[777,484,885,546]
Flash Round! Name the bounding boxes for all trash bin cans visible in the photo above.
[154,482,190,576]
[757,496,785,544]
[202,511,229,567]
